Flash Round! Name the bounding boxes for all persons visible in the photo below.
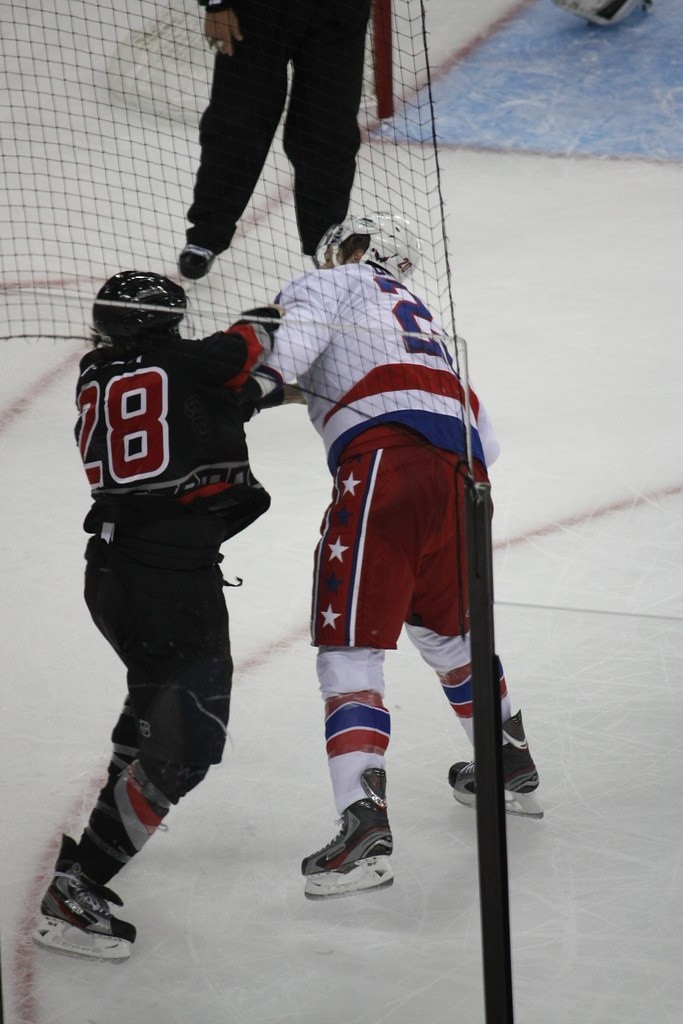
[178,0,373,279]
[35,270,287,957]
[238,209,544,901]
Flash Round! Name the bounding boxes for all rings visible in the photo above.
[217,41,223,47]
[208,37,213,41]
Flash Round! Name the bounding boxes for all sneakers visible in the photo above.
[449,708,544,816]
[33,832,136,956]
[177,243,216,291]
[302,768,392,897]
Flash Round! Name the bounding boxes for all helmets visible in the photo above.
[316,214,421,282]
[93,270,186,346]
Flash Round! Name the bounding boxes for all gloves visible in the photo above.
[243,304,281,332]
